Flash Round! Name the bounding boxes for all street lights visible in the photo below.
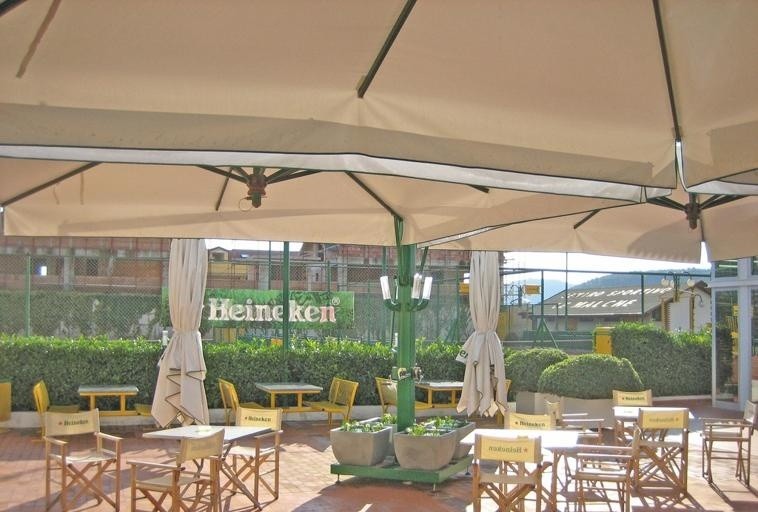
[661,271,695,301]
[689,292,704,336]
[508,285,522,306]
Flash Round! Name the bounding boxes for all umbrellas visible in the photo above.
[149,238,215,471]
[456,251,509,429]
